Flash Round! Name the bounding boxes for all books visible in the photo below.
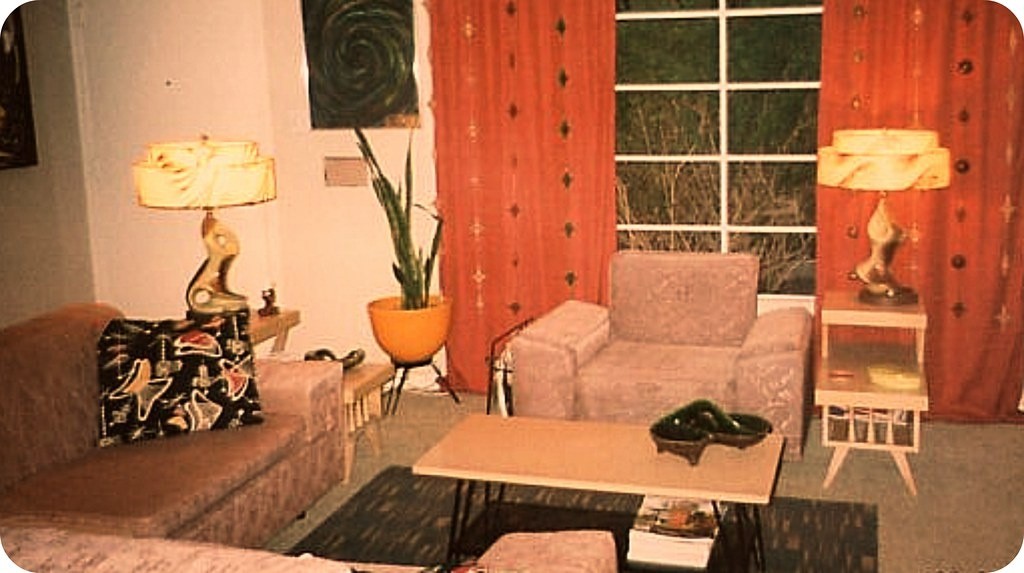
[626,496,728,567]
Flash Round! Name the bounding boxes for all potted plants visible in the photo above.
[347,113,452,362]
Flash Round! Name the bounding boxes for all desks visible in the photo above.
[412,415,788,572]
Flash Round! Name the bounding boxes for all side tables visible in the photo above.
[814,292,929,497]
[246,309,395,434]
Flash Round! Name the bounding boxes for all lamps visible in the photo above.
[131,138,278,322]
[815,128,952,307]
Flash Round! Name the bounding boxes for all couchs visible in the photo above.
[0,301,349,547]
[509,248,815,460]
[0,524,612,573]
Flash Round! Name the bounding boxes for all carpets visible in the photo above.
[280,465,880,572]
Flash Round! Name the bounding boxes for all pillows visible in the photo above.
[94,307,265,447]
